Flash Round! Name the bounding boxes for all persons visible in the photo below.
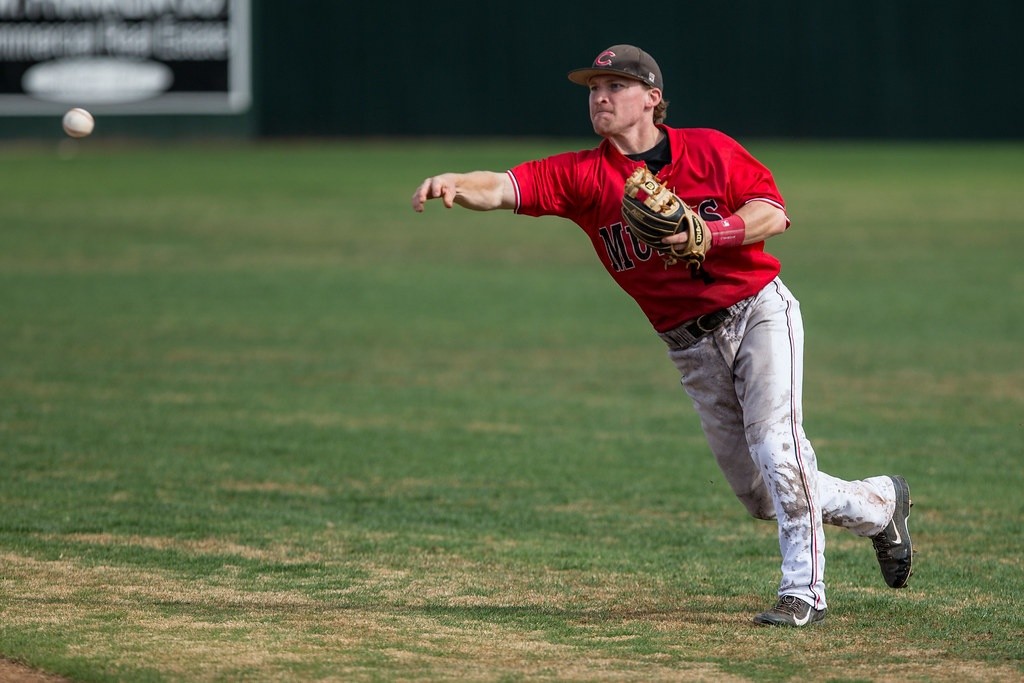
[412,44,915,626]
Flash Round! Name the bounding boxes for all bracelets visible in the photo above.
[706,214,745,247]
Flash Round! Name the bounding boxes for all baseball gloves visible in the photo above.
[622,166,707,263]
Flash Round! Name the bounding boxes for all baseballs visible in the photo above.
[62,108,95,138]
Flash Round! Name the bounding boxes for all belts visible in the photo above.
[686,309,730,338]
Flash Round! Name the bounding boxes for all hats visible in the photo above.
[568,45,663,91]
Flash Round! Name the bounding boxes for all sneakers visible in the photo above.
[869,475,912,588]
[754,596,827,628]
